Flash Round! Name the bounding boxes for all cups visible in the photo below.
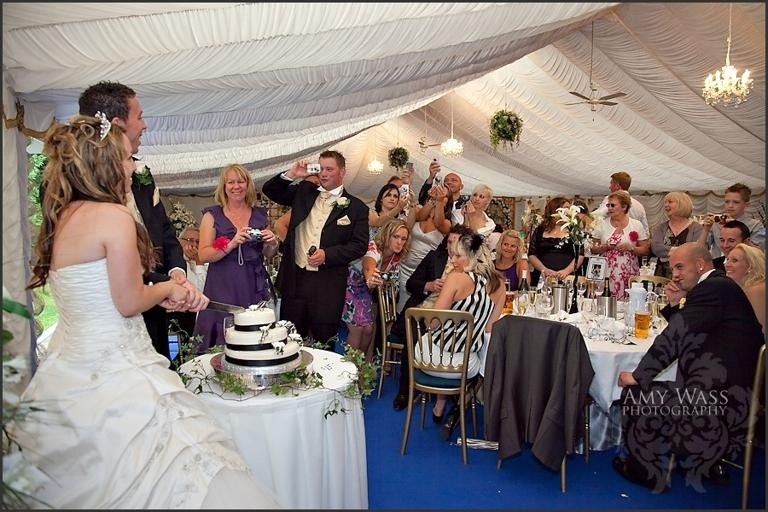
[503,270,677,339]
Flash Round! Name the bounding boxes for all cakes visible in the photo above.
[221,303,303,392]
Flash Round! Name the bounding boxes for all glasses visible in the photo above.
[179,236,200,244]
[606,203,622,207]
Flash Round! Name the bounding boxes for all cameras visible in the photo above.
[434,176,442,187]
[401,184,410,197]
[433,156,438,163]
[306,164,321,174]
[460,194,470,201]
[380,273,391,282]
[714,214,724,222]
[247,228,262,241]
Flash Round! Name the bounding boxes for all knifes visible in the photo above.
[207,301,244,313]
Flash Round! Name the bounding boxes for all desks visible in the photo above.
[175,344,370,510]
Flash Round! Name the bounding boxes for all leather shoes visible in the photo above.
[432,401,445,424]
[676,460,729,483]
[441,404,462,439]
[611,455,668,492]
[392,391,411,412]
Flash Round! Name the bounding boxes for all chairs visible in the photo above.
[363,266,767,511]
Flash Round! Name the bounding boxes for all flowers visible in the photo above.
[489,197,512,231]
[387,146,410,172]
[488,110,526,157]
[1,285,70,511]
[210,236,232,256]
[329,194,352,212]
[163,197,195,239]
[628,229,640,242]
[130,157,152,190]
[519,206,546,229]
[549,202,607,255]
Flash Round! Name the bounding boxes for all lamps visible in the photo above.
[700,1,757,110]
[364,126,387,176]
[441,91,464,159]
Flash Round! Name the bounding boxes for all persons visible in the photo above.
[261,150,370,352]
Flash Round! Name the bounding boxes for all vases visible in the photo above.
[529,225,534,243]
[569,243,582,314]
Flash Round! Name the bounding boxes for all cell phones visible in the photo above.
[406,162,412,170]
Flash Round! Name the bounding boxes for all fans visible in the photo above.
[416,106,440,154]
[562,21,629,113]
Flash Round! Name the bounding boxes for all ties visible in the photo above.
[318,191,331,207]
